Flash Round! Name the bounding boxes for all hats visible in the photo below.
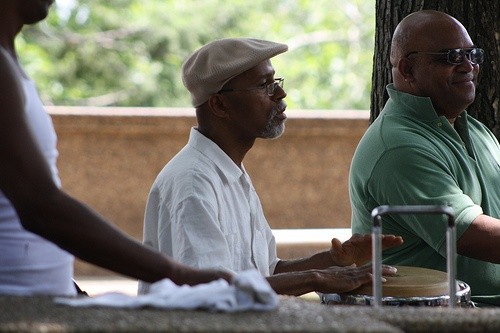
[182,39,288,108]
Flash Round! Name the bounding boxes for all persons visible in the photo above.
[350,9,500,306]
[0,0,234,298]
[136,37,404,306]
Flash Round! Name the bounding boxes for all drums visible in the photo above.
[315,263,473,309]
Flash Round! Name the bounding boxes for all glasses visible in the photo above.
[405,47,486,65]
[225,78,284,96]
